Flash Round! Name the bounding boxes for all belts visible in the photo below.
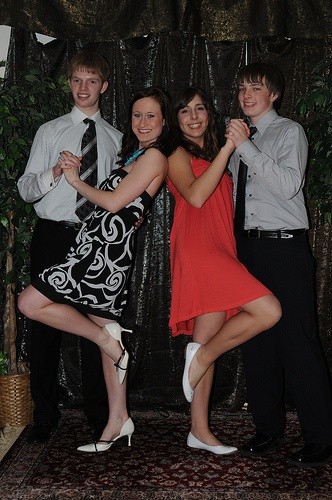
[243,228,307,239]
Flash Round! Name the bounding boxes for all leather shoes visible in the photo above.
[288,443,332,467]
[243,432,278,456]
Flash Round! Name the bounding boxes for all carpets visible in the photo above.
[0,409,332,500]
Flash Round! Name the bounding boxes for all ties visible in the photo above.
[76,118,99,222]
[234,127,257,236]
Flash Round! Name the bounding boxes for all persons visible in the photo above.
[164,86,283,456]
[18,87,168,452]
[16,51,123,444]
[222,62,332,466]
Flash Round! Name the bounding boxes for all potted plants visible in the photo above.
[0,75,75,428]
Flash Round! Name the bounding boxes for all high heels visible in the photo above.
[76,418,135,453]
[105,323,133,385]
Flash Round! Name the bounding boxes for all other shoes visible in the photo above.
[186,432,238,456]
[182,342,201,403]
